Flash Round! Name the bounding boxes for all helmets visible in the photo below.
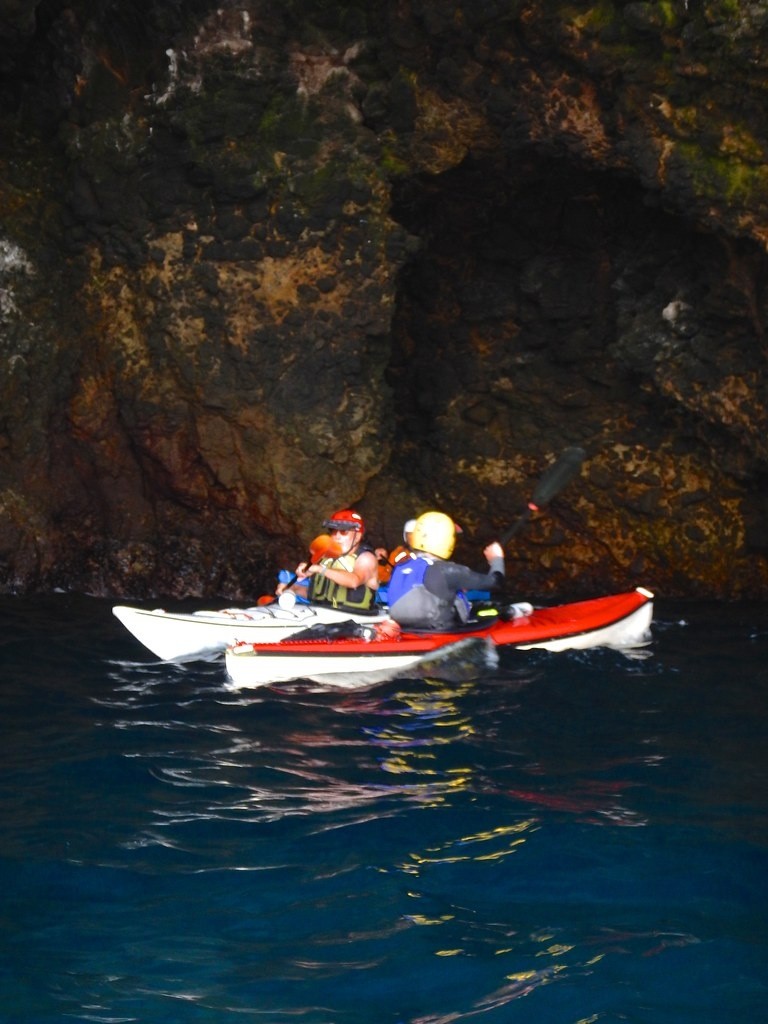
[404,511,463,559]
[323,510,364,532]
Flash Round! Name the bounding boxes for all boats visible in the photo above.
[225,588,657,683]
[112,602,390,662]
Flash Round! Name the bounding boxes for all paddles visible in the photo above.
[255,534,343,606]
[381,554,395,571]
[479,448,586,570]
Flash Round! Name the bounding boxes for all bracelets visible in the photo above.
[321,564,327,575]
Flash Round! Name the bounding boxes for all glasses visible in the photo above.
[330,529,355,537]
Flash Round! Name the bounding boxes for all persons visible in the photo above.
[275,510,379,615]
[375,519,414,583]
[389,512,533,628]
